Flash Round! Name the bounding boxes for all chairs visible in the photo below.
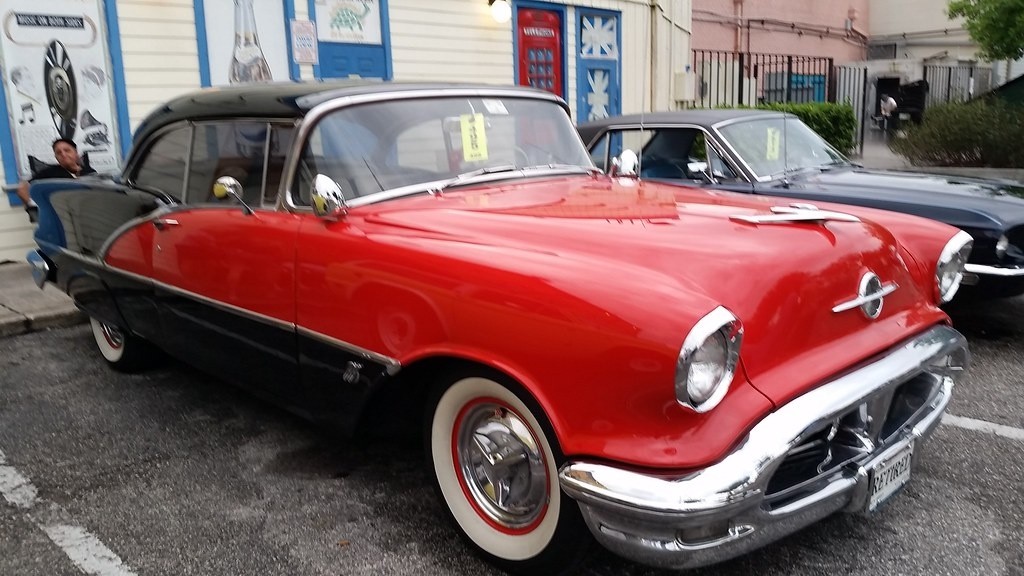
[643,152,685,182]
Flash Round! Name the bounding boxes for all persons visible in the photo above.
[880,90,898,146]
[17,138,97,204]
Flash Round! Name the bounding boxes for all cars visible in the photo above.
[571,108,1024,304]
[26,83,969,575]
